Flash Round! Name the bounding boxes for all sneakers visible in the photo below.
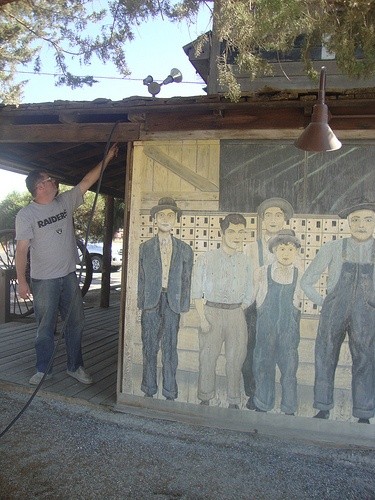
[66,365,93,384]
[29,371,53,385]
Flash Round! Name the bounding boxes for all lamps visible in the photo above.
[294,67,342,151]
[142,68,183,97]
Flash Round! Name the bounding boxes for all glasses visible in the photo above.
[41,177,55,184]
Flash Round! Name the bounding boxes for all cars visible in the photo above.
[76,236,124,273]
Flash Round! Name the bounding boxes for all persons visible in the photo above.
[13,142,123,384]
